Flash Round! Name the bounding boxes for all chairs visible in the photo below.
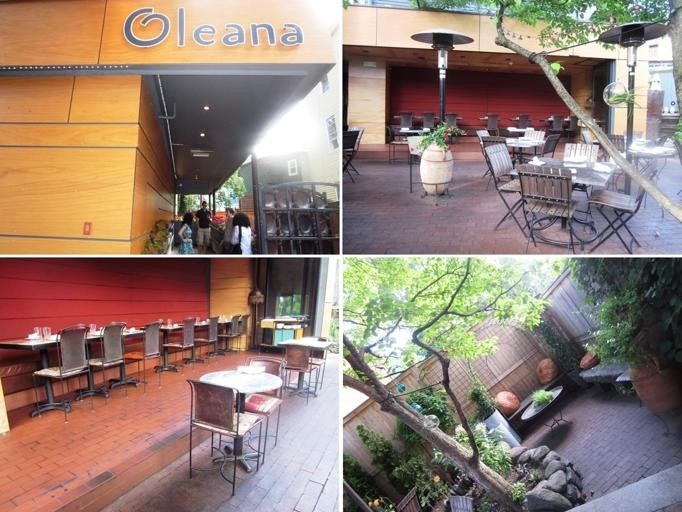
[343,113,676,255]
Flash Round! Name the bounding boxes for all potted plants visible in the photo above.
[579,287,682,417]
[530,389,554,413]
[469,388,521,444]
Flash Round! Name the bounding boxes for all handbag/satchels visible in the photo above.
[223,241,243,255]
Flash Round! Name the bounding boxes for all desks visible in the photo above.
[520,385,574,440]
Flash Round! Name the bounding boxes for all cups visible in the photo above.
[167,318,172,328]
[34,327,41,339]
[90,324,96,336]
[43,327,51,339]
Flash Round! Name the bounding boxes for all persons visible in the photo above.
[195,201,212,254]
[224,207,235,254]
[178,213,194,254]
[232,213,253,254]
